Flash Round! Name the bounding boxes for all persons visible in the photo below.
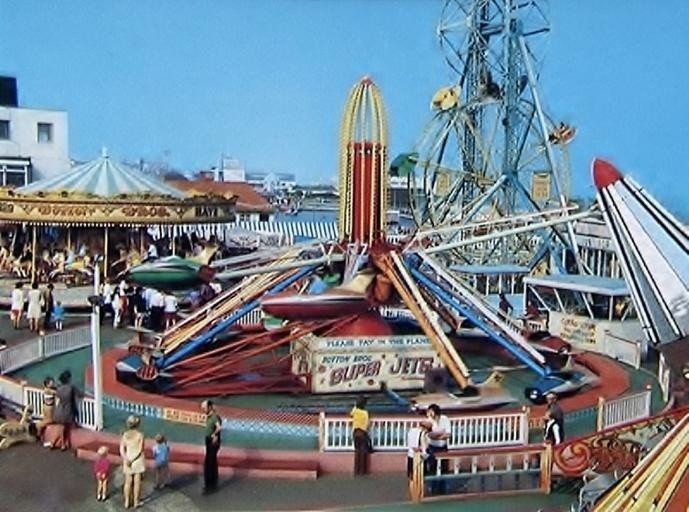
[426,404,451,496]
[25,283,40,331]
[0,225,220,275]
[37,375,55,438]
[97,274,224,333]
[55,369,93,451]
[540,409,561,451]
[118,414,147,509]
[523,299,538,321]
[495,293,514,322]
[150,432,170,490]
[199,398,222,496]
[40,284,53,328]
[406,420,432,503]
[615,297,626,316]
[94,445,110,503]
[545,391,565,447]
[349,394,376,479]
[52,298,64,331]
[8,280,25,331]
